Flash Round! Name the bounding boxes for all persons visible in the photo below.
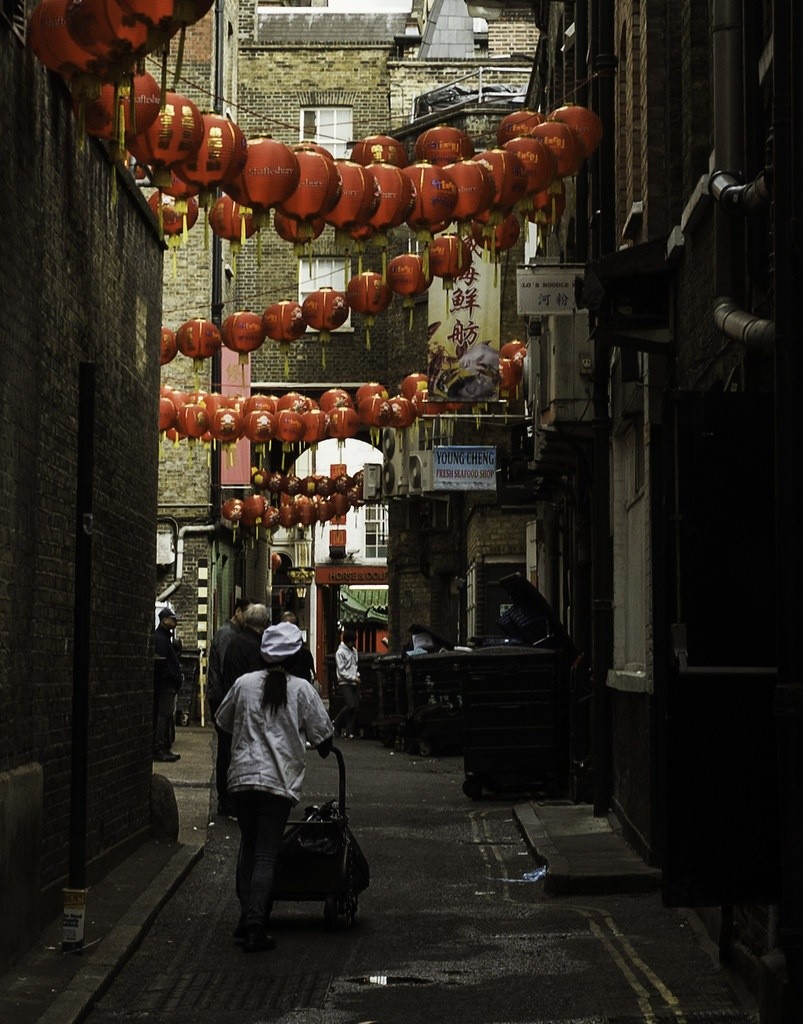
[153,597,364,950]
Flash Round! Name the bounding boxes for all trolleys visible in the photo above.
[234,745,357,934]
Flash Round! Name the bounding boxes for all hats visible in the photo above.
[159,607,182,618]
[260,622,303,663]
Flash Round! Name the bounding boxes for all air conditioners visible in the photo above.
[363,427,433,500]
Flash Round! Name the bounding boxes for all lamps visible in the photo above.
[329,546,346,559]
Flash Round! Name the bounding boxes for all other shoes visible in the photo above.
[151,752,181,762]
[233,920,250,939]
[244,935,268,953]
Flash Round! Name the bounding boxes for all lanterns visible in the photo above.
[27,0,599,571]
